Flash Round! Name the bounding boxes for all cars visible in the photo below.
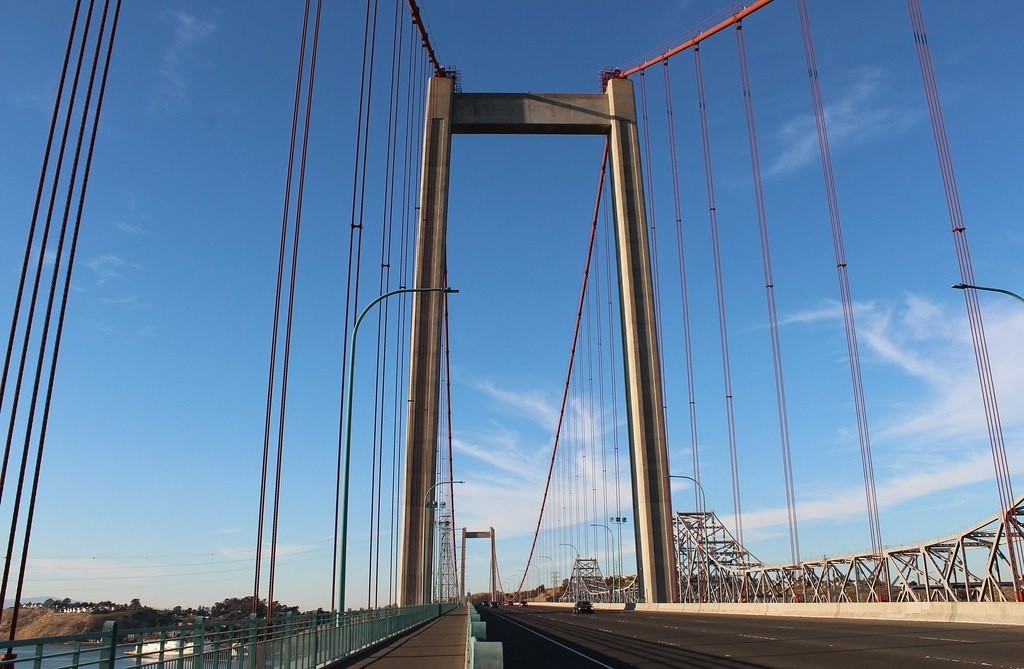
[490,602,498,609]
[506,600,513,606]
[519,600,528,608]
[572,601,595,615]
[482,601,488,606]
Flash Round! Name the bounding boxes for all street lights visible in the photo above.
[338,286,461,625]
[510,522,625,607]
[422,480,467,603]
